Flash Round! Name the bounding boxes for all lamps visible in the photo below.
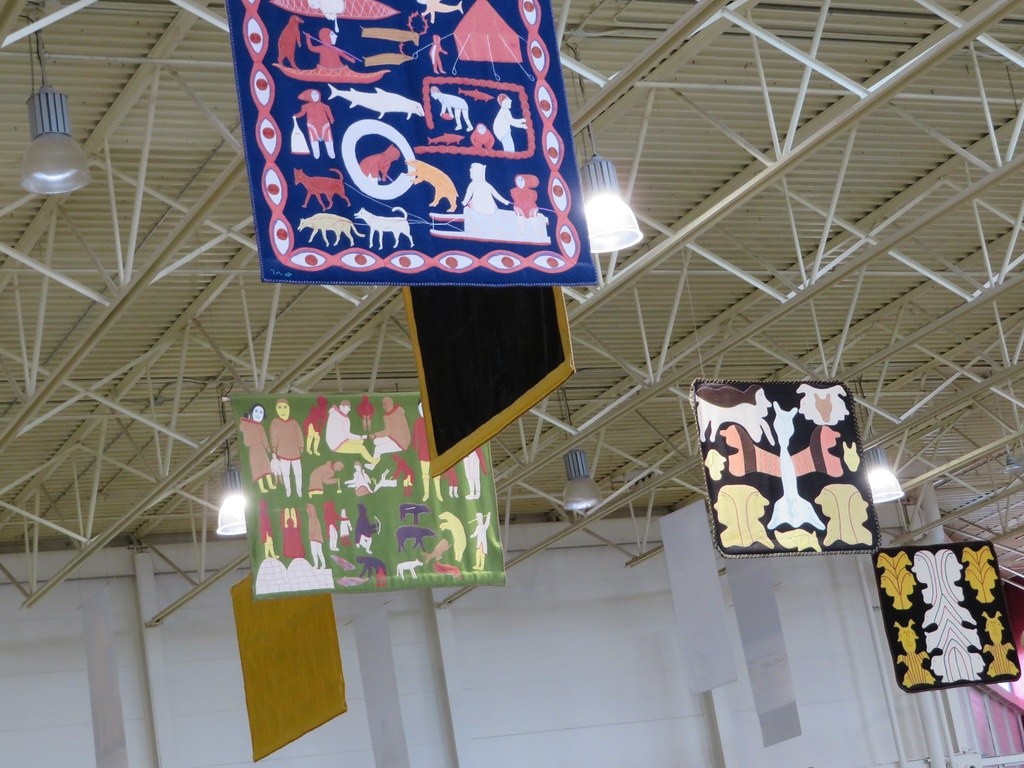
[854,378,905,505]
[22,14,92,194]
[566,41,644,253]
[557,385,604,510]
[216,382,252,536]
